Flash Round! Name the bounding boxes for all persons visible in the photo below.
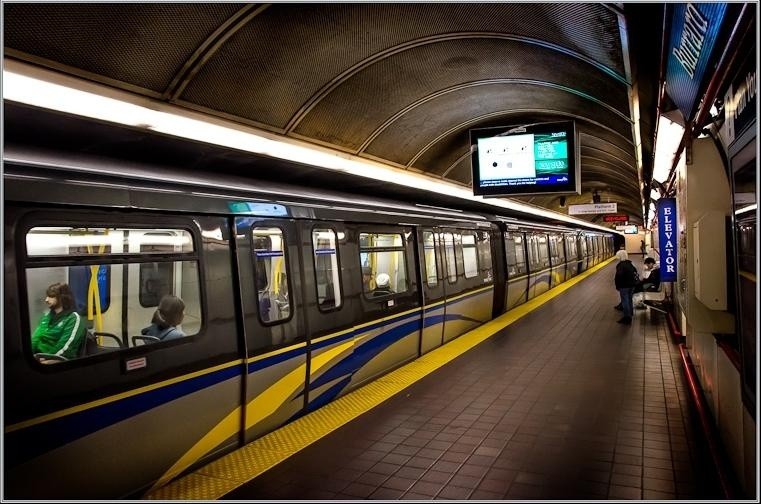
[31,282,86,363]
[616,250,637,326]
[373,273,395,297]
[614,257,660,312]
[141,295,187,345]
[640,240,645,258]
[362,272,376,298]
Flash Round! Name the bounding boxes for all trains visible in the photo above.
[733,205,757,377]
[2,161,626,501]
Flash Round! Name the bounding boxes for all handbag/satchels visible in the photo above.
[633,272,645,294]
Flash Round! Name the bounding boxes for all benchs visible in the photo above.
[643,282,672,320]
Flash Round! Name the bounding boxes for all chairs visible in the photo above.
[35,327,160,365]
[371,291,391,297]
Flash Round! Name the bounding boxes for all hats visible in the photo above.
[376,273,390,289]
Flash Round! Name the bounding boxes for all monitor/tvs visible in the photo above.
[624,224,638,234]
[470,120,582,198]
